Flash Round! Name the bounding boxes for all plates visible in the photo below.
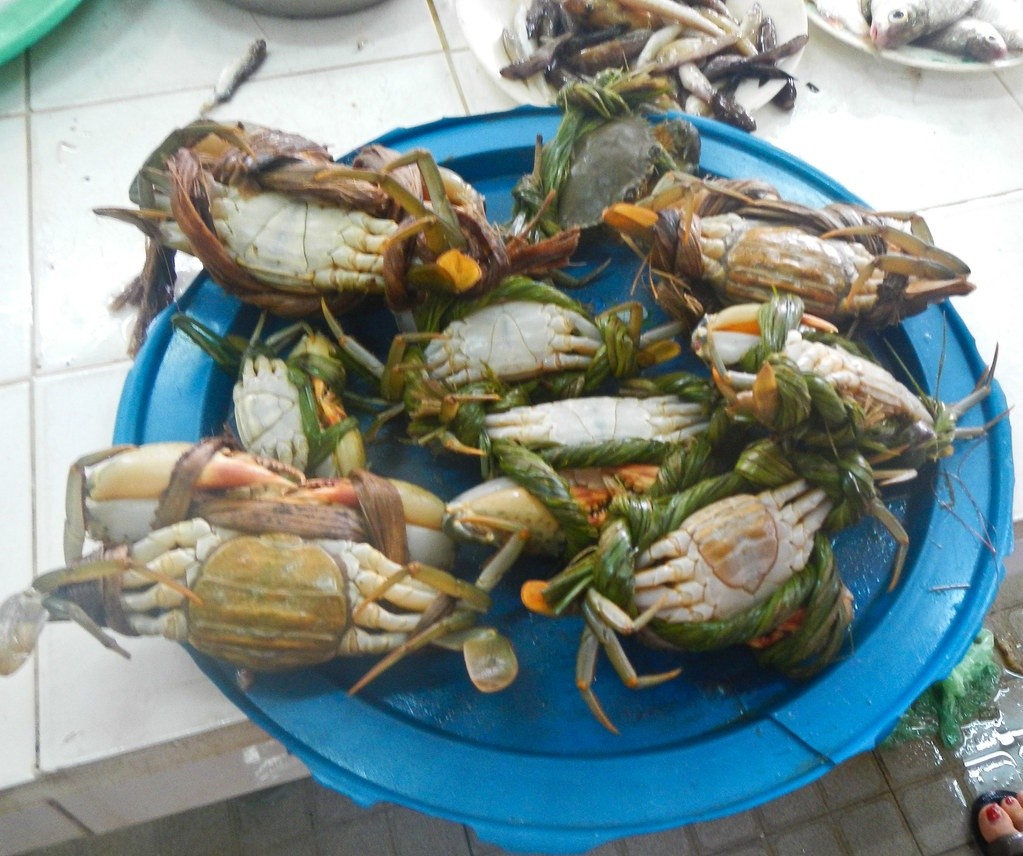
[0,0,80,68]
[456,0,810,118]
[110,105,1015,856]
[805,0,1023,72]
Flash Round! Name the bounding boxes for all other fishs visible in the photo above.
[499,0,1023,133]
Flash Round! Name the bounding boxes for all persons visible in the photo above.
[971,790,1023,856]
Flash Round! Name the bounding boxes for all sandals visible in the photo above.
[972,789,1023,856]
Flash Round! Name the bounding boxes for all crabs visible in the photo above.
[1,64,1016,736]
